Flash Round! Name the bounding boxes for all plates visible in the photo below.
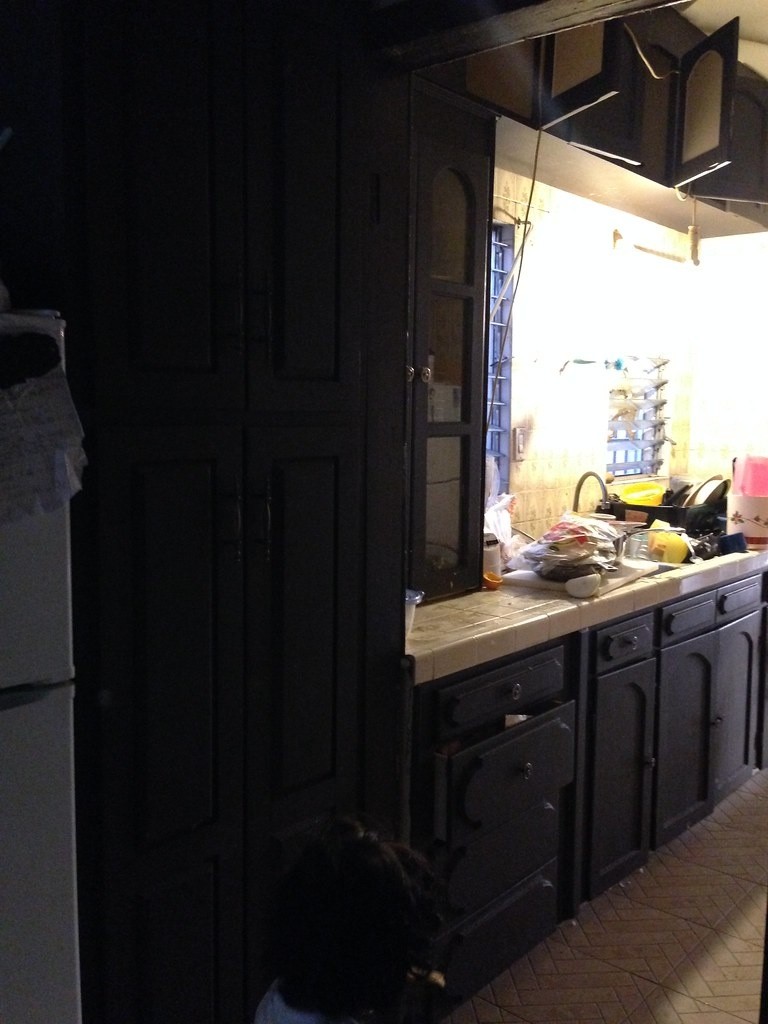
[682,474,723,505]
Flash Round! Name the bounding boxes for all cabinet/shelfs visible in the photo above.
[0,0,768,1024]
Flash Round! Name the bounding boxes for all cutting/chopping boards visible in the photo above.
[501,556,659,597]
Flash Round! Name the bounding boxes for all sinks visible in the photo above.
[639,564,680,579]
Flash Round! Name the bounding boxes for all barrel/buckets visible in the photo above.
[726,497,768,550]
[732,453,768,497]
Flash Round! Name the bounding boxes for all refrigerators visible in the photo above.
[0,311,82,1024]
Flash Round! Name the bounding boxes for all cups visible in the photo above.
[405,588,425,640]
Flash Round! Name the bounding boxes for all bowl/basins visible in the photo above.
[693,478,732,505]
[531,553,618,582]
[621,483,664,506]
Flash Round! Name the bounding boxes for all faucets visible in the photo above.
[571,470,611,514]
